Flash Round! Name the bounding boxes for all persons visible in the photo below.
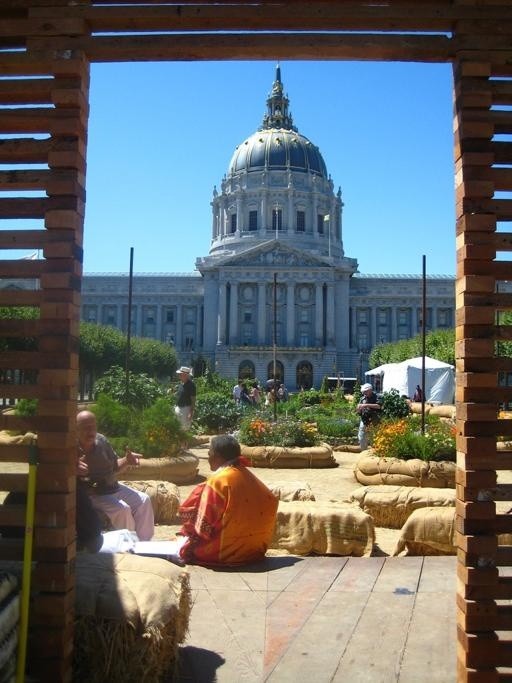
[174,366,197,431]
[249,382,262,416]
[4,448,104,555]
[239,383,249,408]
[77,410,155,540]
[233,379,243,405]
[413,385,427,402]
[175,433,280,567]
[356,382,385,450]
[310,386,315,391]
[264,384,289,409]
[304,384,310,390]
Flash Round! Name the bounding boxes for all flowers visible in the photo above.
[231,409,459,460]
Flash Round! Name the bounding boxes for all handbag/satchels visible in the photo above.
[360,408,380,426]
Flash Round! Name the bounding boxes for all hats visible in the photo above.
[175,366,193,376]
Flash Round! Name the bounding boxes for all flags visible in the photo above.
[323,214,330,221]
